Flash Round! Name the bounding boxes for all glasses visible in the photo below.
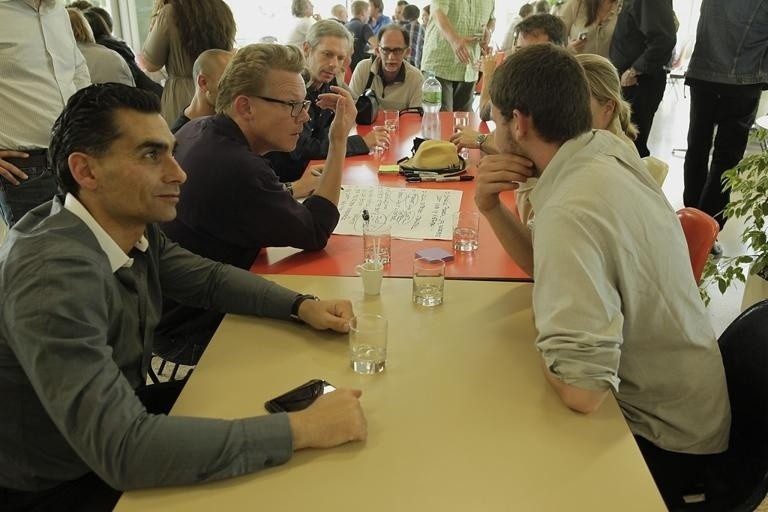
[399,106,425,119]
[246,94,312,119]
[379,43,409,56]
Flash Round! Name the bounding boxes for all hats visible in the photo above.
[400,138,468,180]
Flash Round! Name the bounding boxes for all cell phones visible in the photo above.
[265,379,336,414]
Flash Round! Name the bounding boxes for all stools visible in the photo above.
[145,350,194,386]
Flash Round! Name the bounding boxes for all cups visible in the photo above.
[452,110,468,133]
[371,124,390,151]
[384,108,399,133]
[450,206,482,254]
[457,146,468,158]
[360,221,391,273]
[347,311,388,376]
[354,260,384,299]
[411,256,446,309]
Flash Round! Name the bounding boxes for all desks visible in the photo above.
[110,272,672,512]
[247,108,530,282]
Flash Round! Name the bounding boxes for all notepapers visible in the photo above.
[379,165,400,173]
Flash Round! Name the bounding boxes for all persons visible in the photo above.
[0,83,367,511]
[473,44,732,502]
[138,41,359,414]
[0,1,768,255]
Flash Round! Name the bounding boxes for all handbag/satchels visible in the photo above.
[356,86,380,126]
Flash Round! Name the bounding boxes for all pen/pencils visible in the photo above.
[311,168,345,190]
[399,170,474,182]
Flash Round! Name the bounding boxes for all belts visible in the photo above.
[1,151,52,170]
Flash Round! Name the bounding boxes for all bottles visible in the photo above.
[420,69,442,143]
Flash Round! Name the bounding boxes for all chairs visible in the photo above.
[675,205,718,281]
[644,155,669,190]
[664,301,767,512]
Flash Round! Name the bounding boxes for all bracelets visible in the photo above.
[290,294,314,322]
